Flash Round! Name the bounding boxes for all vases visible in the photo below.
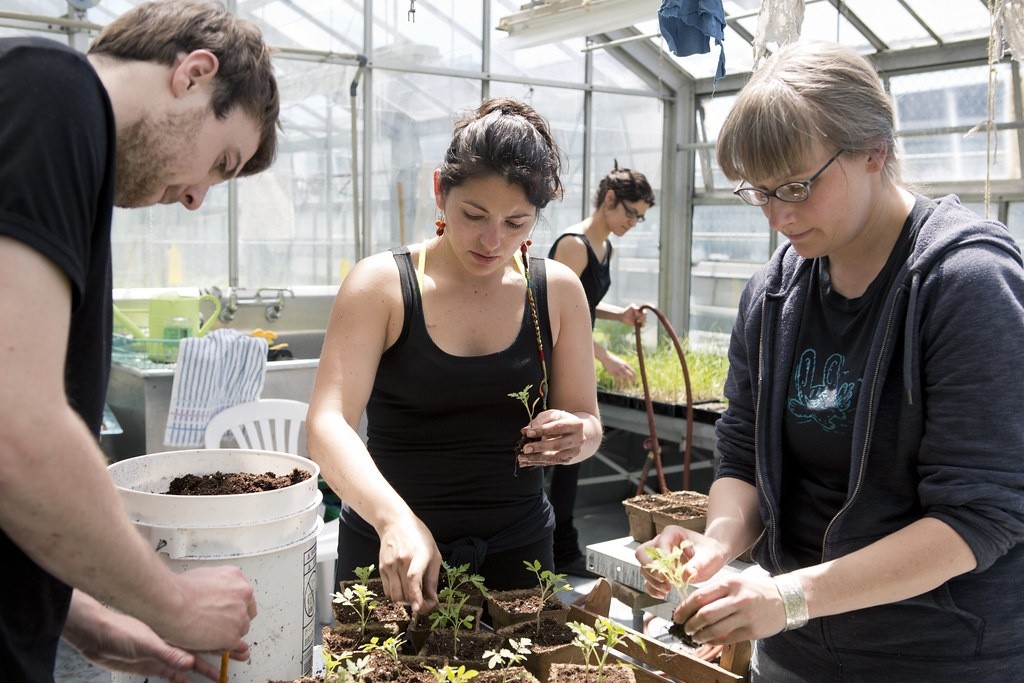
[621,491,756,563]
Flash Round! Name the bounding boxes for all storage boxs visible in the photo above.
[113,324,181,370]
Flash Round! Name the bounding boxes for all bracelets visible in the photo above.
[772,572,810,633]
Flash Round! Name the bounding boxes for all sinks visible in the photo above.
[100,329,368,481]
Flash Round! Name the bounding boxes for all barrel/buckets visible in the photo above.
[106,451,324,683]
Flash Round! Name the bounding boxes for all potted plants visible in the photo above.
[295,559,588,683]
[550,615,647,683]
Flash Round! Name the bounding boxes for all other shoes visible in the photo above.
[554,548,605,578]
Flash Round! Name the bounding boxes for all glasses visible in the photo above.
[733,149,845,207]
[620,199,645,223]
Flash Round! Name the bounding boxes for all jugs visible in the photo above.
[113,294,222,363]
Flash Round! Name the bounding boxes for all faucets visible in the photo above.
[219,287,294,323]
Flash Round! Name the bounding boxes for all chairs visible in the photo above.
[205,398,339,624]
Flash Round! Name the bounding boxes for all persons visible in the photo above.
[1,0,283,682]
[637,40,1019,683]
[549,157,658,582]
[303,94,605,614]
